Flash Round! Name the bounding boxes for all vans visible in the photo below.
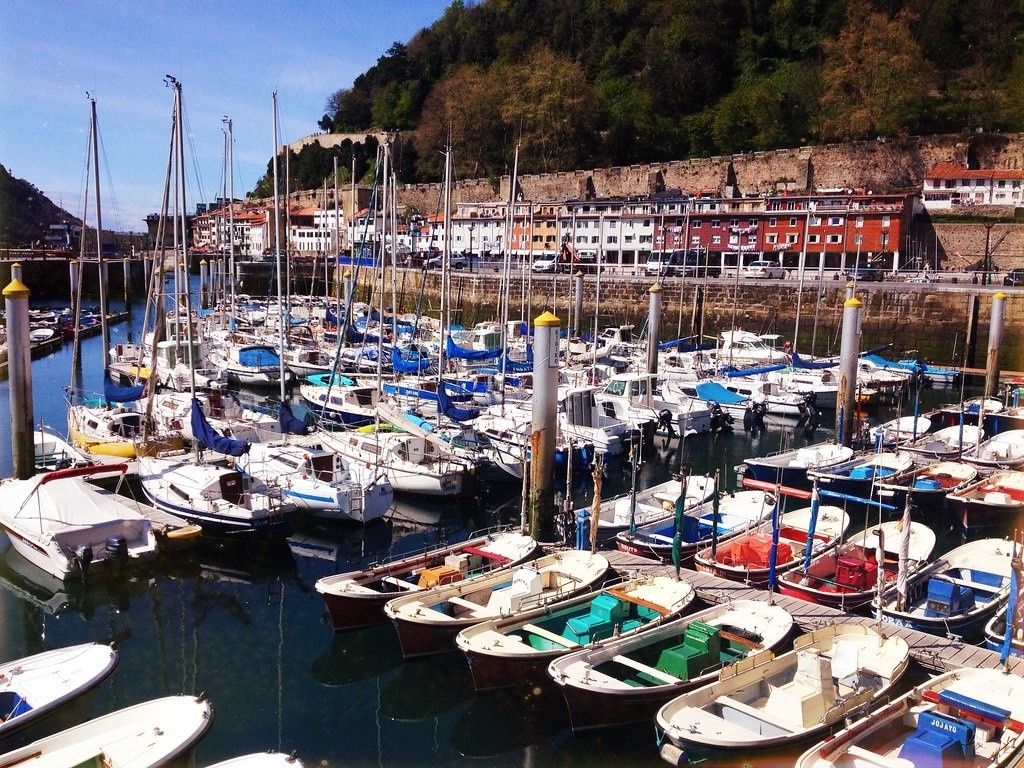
[531,252,602,275]
[645,249,722,278]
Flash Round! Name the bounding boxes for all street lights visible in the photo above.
[468,225,474,275]
[879,230,891,285]
[980,219,992,284]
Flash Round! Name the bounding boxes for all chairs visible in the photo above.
[417,555,467,588]
[896,710,977,768]
[656,620,721,681]
[924,579,976,619]
[563,595,623,644]
[486,569,542,614]
[751,533,793,567]
[835,556,877,593]
[765,648,836,728]
[675,515,714,544]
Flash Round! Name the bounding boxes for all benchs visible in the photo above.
[381,493,1011,768]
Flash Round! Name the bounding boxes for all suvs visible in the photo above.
[400,251,465,268]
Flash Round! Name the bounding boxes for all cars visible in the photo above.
[847,265,882,280]
[743,261,786,279]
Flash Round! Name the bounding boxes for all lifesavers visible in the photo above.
[183,325,196,336]
[784,341,794,354]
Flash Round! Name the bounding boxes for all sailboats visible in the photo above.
[0,74,963,533]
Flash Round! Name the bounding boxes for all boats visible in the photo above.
[0,691,213,768]
[0,642,121,741]
[316,312,1024,768]
[1,262,203,579]
